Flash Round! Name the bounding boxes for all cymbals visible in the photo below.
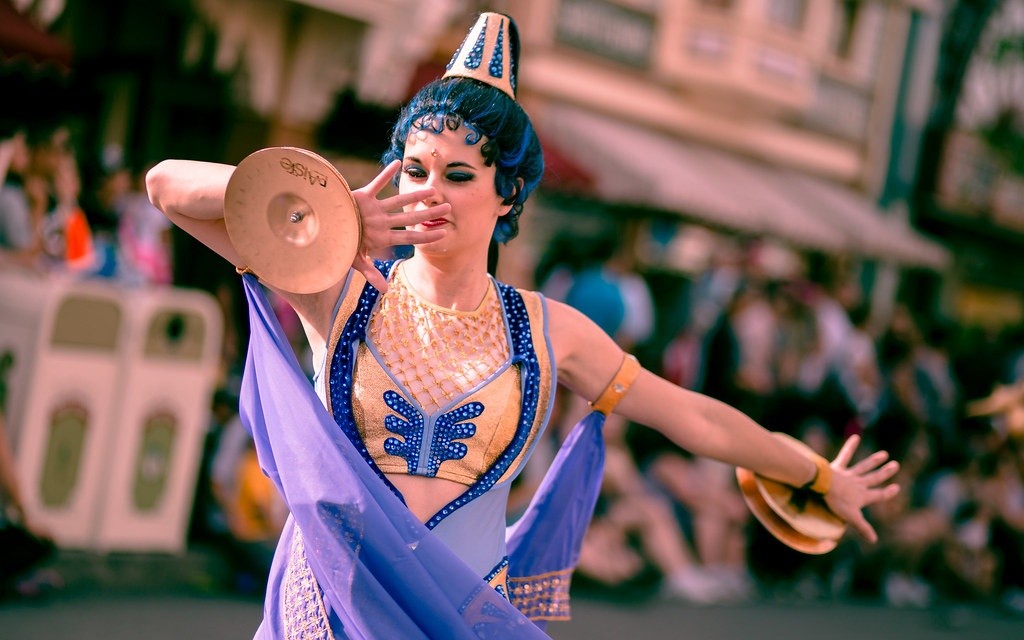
[221,143,364,297]
[734,430,850,557]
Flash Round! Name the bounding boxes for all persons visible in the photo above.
[145,12,901,639]
[536,201,1023,620]
[1,101,173,284]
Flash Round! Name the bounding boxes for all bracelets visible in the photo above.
[806,449,832,494]
